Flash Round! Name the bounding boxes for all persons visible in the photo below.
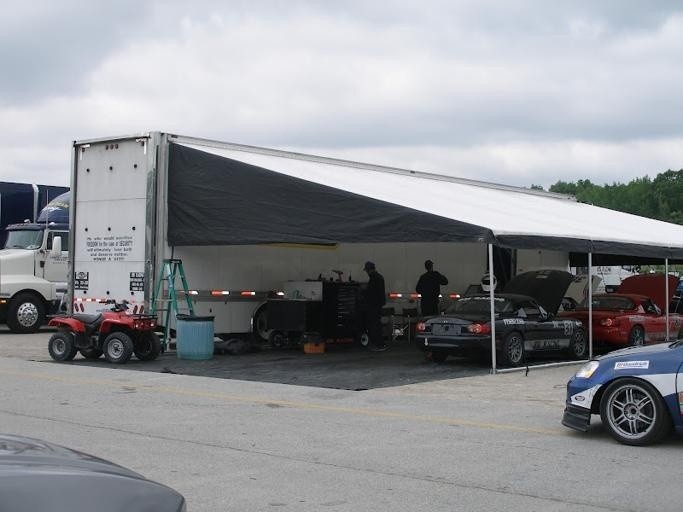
[415,259,449,352]
[356,260,388,352]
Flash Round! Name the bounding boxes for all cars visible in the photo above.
[561,339,683,447]
[1,430,186,512]
[414,268,683,367]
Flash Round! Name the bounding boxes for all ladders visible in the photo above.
[147,259,199,354]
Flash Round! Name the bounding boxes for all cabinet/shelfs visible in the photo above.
[304,280,369,345]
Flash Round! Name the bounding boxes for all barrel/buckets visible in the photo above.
[175,313,216,361]
[302,331,325,354]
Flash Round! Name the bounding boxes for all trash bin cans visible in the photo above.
[175,316,216,359]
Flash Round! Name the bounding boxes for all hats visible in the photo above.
[363,261,375,271]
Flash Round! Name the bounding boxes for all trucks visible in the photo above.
[0,183,71,334]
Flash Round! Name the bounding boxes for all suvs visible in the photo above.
[46,300,162,364]
